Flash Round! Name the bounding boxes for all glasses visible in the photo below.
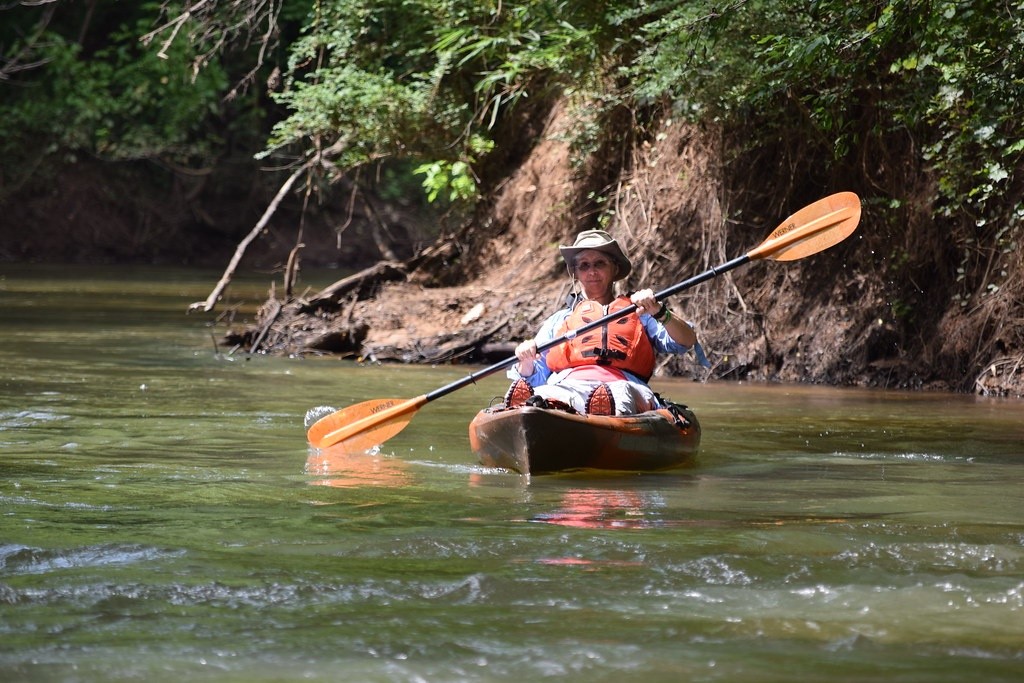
[575,260,607,272]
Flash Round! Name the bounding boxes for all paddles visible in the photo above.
[307,191,861,455]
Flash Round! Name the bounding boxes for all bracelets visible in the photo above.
[651,301,671,326]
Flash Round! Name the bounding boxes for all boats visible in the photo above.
[466,391,702,483]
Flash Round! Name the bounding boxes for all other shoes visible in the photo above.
[506,378,534,407]
[584,382,616,416]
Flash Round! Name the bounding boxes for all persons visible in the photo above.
[505,229,696,416]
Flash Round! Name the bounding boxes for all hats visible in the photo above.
[559,230,631,282]
